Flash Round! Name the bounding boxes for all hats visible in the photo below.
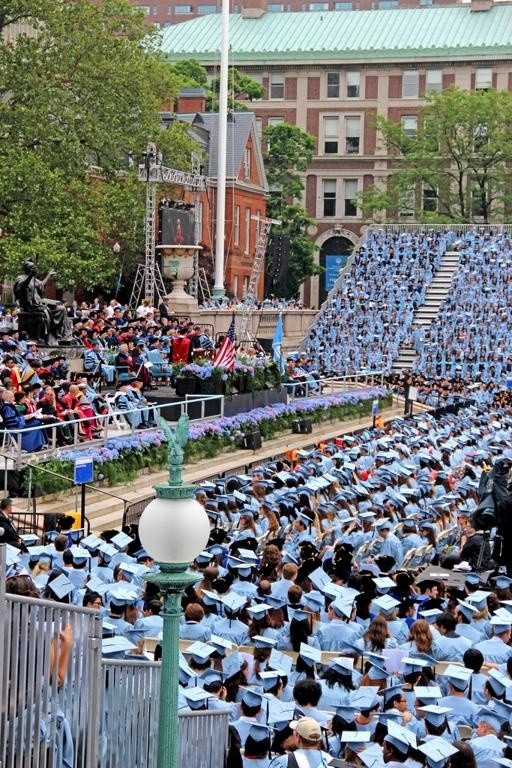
[0,330,67,402]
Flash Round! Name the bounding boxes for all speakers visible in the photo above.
[265,235,289,286]
[243,431,261,450]
[292,419,312,433]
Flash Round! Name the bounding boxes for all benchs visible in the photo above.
[304,232,512,381]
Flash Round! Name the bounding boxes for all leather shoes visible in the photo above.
[35,427,102,450]
[136,422,157,429]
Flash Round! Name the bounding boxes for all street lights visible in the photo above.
[136,407,213,767]
[111,236,128,257]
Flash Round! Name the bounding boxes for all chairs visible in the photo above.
[83,339,174,391]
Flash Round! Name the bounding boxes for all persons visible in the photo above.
[0,223,512,766]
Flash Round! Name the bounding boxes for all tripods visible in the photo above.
[476,533,504,569]
